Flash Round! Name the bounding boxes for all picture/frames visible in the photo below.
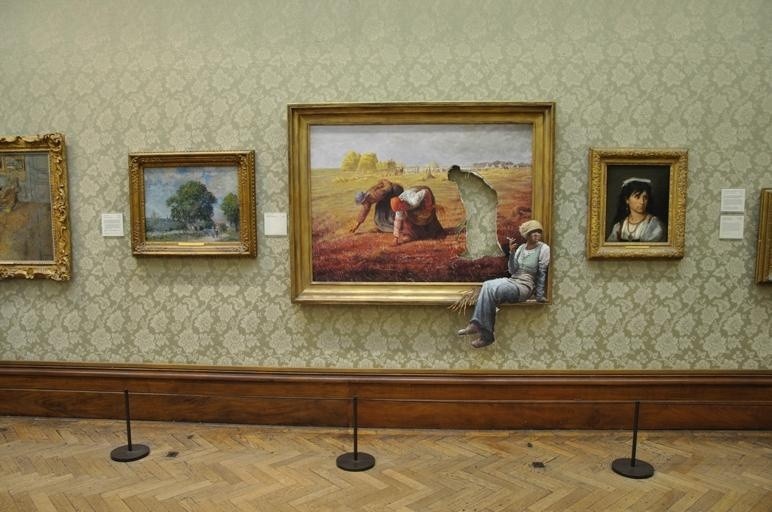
[586,148,690,261]
[0,131,72,283]
[287,102,556,308]
[128,150,258,261]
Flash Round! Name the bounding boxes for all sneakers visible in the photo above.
[471,337,488,348]
[457,323,479,335]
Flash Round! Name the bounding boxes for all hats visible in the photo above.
[390,197,400,212]
[355,191,370,206]
[519,220,543,239]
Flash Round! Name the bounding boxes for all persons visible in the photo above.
[389,186,447,245]
[446,164,505,258]
[348,178,404,234]
[456,219,553,349]
[605,177,667,243]
